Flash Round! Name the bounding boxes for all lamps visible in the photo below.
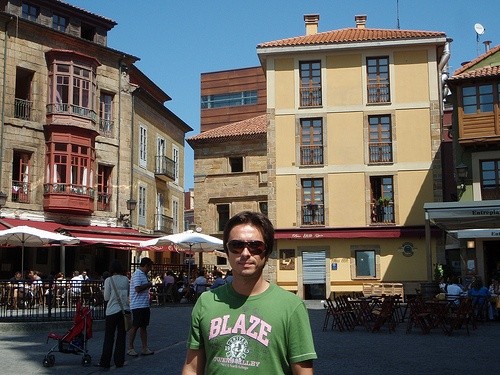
[454,161,470,193]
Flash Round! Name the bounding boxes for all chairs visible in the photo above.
[321,289,404,334]
[404,292,489,338]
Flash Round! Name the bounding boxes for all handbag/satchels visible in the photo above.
[124,312,132,331]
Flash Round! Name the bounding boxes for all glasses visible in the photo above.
[226,240,266,255]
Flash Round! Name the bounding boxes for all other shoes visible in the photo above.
[124,361,128,366]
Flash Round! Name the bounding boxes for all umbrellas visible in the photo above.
[140,229,224,281]
[0,226,81,281]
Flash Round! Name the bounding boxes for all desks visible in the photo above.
[347,297,374,333]
[436,291,500,324]
[422,297,457,335]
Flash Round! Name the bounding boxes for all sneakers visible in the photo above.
[126,348,138,356]
[140,347,154,355]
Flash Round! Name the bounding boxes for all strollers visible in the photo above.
[42,295,99,367]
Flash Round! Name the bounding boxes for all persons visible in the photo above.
[129,257,156,356]
[182,210,318,375]
[8,270,108,309]
[438,274,500,323]
[149,267,233,305]
[94,260,129,368]
